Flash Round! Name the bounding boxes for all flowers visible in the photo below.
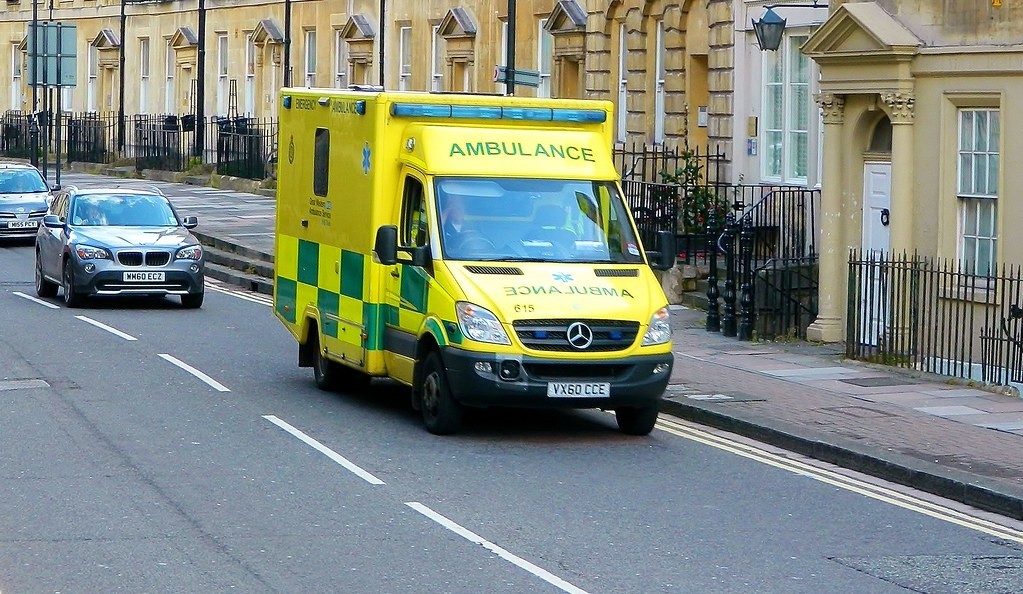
[656,149,729,230]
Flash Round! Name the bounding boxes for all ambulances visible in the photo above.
[274,82,674,436]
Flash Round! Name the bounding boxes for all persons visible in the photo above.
[442,196,476,249]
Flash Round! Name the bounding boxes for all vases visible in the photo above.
[677,233,718,254]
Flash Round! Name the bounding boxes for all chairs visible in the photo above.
[526,205,572,248]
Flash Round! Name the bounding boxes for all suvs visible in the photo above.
[34,185,204,306]
[0,161,61,239]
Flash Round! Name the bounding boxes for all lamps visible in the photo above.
[750,0,828,52]
